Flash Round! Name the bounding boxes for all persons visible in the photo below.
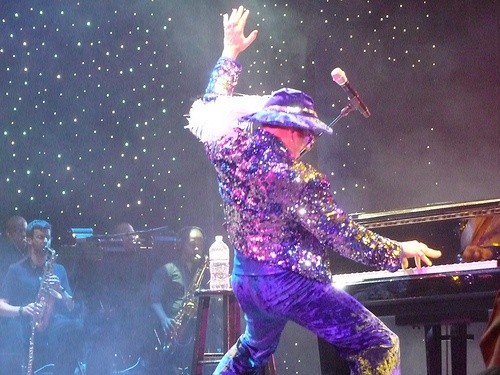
[0,220,74,375]
[149,226,213,373]
[186,6,441,375]
[460,243,500,266]
[101,223,146,296]
[1,216,30,291]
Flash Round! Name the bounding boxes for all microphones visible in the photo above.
[330,67,371,119]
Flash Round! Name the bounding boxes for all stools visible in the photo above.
[190,288,277,375]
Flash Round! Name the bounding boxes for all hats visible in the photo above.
[238,88,334,133]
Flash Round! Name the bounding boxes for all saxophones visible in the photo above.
[155,254,209,360]
[31,246,59,337]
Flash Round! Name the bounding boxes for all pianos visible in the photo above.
[314,199,500,374]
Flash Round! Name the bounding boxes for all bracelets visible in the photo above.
[18,305,23,318]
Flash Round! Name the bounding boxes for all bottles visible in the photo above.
[207,234,230,292]
